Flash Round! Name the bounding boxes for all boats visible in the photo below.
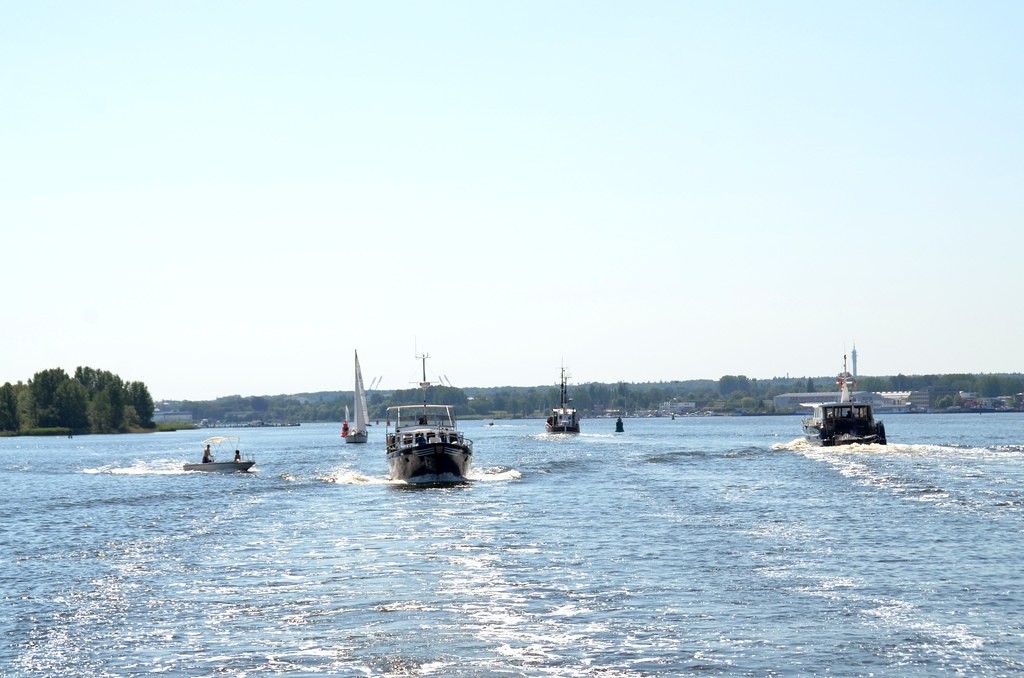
[799,354,886,448]
[182,434,256,473]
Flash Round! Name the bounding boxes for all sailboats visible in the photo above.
[545,359,581,434]
[341,348,373,444]
[384,352,474,478]
